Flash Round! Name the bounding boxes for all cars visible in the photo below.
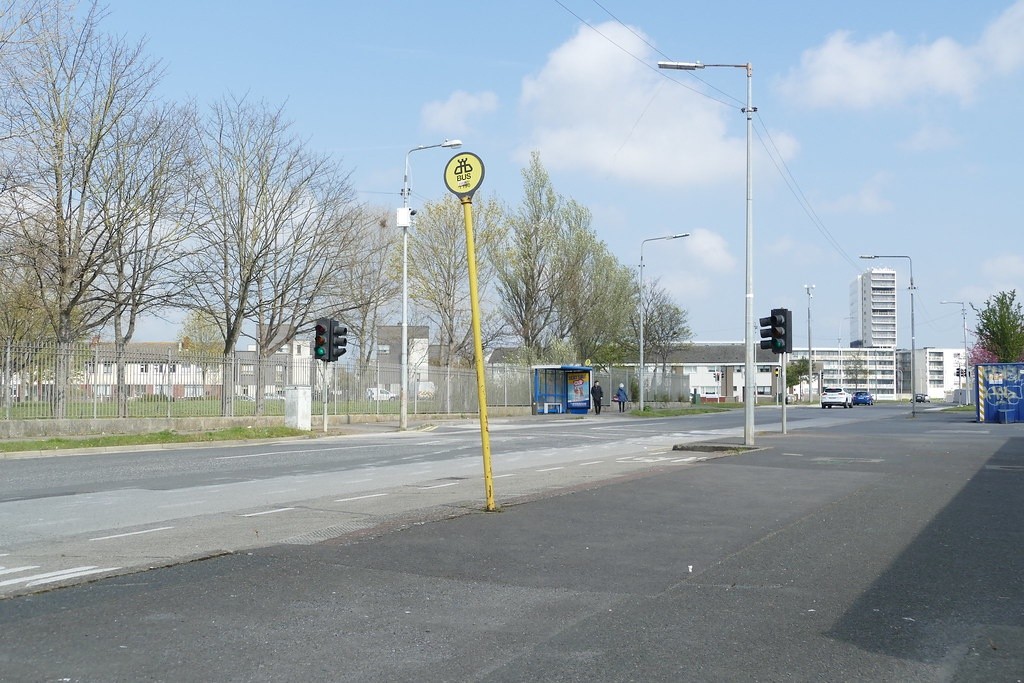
[910,394,931,403]
[854,391,874,406]
[126,389,345,401]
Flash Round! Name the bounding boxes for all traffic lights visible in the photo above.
[964,369,966,376]
[956,368,960,377]
[774,367,779,379]
[770,308,788,354]
[713,373,716,378]
[961,368,964,377]
[759,316,773,350]
[821,372,823,380]
[721,373,724,379]
[330,319,347,362]
[314,318,329,359]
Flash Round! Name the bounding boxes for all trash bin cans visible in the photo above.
[996,409,1014,423]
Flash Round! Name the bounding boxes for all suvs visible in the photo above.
[821,387,853,408]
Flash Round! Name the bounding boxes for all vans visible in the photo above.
[366,387,396,403]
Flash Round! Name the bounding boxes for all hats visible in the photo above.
[619,383,624,388]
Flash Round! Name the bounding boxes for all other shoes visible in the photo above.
[596,413,600,415]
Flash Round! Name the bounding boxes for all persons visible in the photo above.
[616,383,628,413]
[591,381,603,415]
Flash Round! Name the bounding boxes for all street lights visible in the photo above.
[399,139,464,429]
[804,284,816,404]
[859,255,916,418]
[638,233,690,409]
[940,301,969,407]
[658,61,755,446]
